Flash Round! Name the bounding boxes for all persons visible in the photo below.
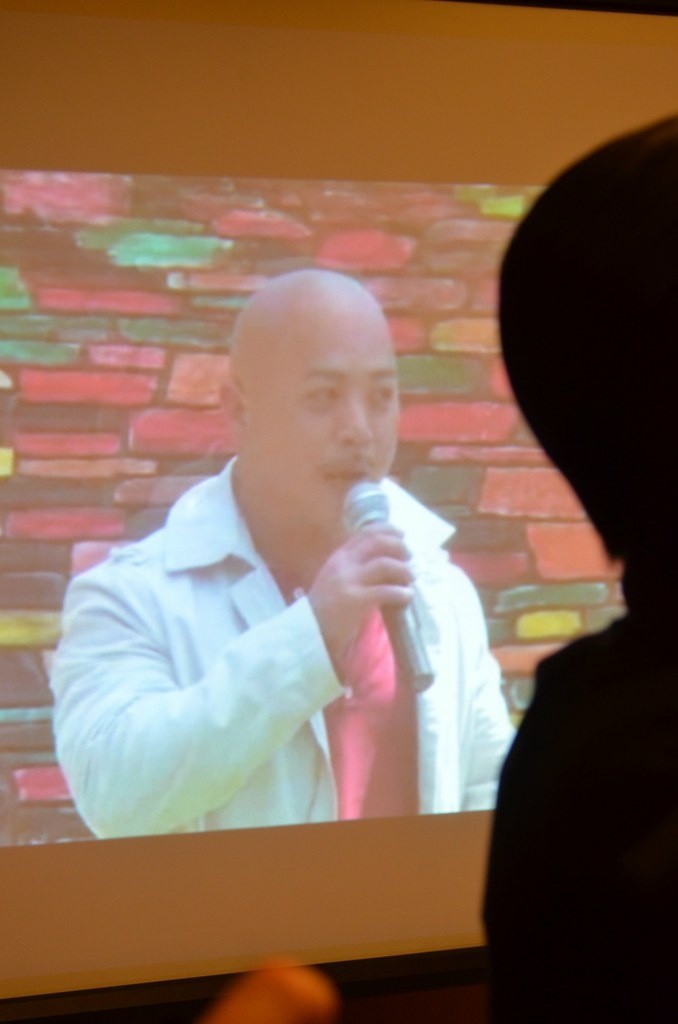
[481,116,678,1024]
[48,270,520,840]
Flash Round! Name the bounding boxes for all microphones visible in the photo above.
[344,483,434,695]
